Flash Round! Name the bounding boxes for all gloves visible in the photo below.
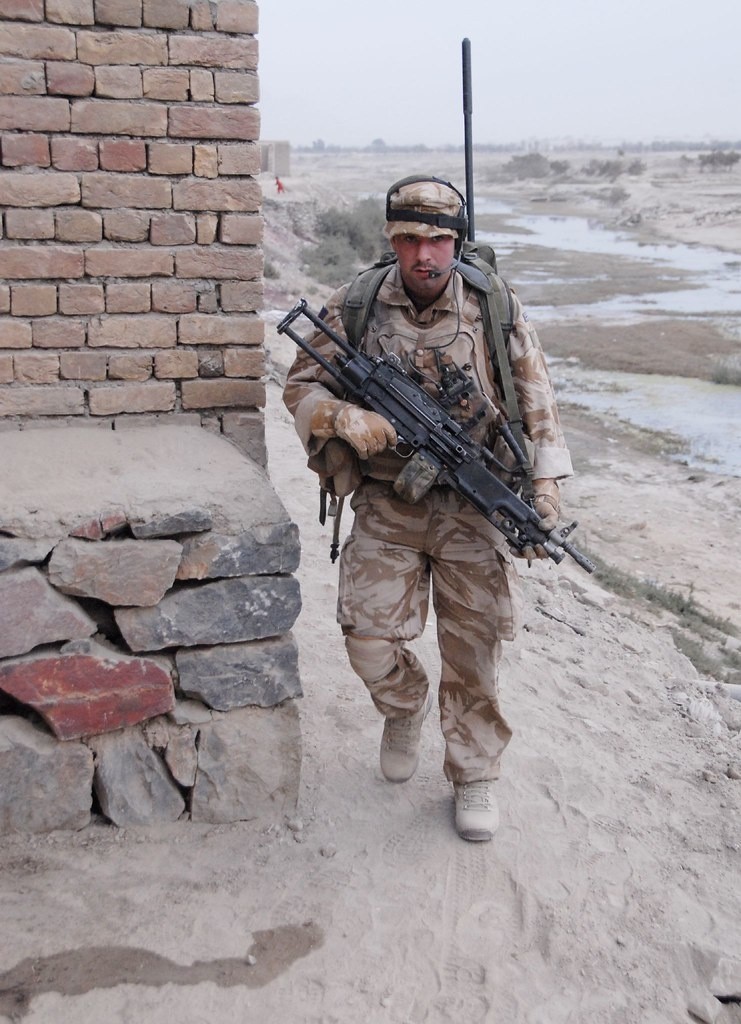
[310,398,397,460]
[509,480,560,559]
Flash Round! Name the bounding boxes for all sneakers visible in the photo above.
[453,779,500,841]
[380,692,432,782]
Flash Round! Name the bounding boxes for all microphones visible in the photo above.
[429,250,461,279]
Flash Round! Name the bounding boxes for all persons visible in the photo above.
[283,174,574,840]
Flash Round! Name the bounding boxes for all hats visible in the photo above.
[381,181,463,238]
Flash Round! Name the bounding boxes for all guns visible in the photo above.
[276,297,597,576]
